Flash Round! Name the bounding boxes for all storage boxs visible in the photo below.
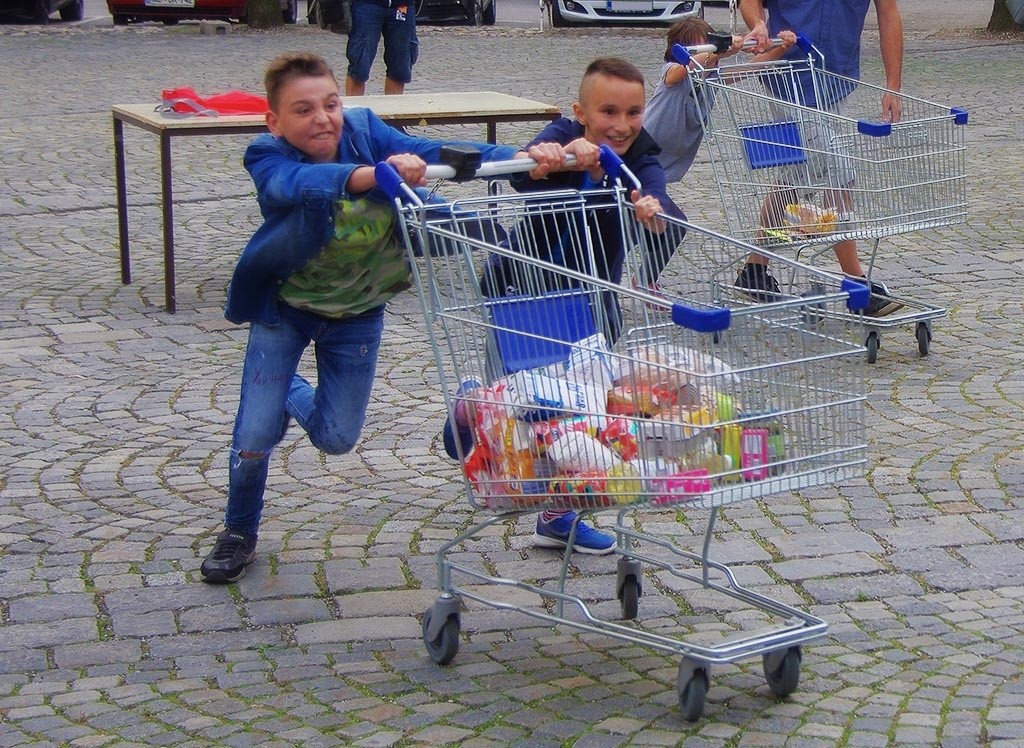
[494,333,767,504]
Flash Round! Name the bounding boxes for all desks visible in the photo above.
[112,92,561,313]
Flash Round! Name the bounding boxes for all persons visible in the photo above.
[199,49,566,583]
[444,57,668,554]
[620,18,798,311]
[345,0,419,137]
[732,0,906,319]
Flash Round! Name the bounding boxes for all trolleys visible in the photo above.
[669,36,970,363]
[375,141,869,724]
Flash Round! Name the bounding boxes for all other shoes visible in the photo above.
[850,281,905,320]
[730,264,786,308]
[630,275,672,312]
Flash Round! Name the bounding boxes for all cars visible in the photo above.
[305,0,497,36]
[545,0,705,29]
[0,0,86,23]
[105,1,297,29]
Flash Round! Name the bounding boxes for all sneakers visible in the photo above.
[533,512,617,555]
[443,377,486,462]
[275,414,293,444]
[200,530,260,583]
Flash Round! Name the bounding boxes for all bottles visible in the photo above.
[546,463,640,506]
[632,454,732,488]
